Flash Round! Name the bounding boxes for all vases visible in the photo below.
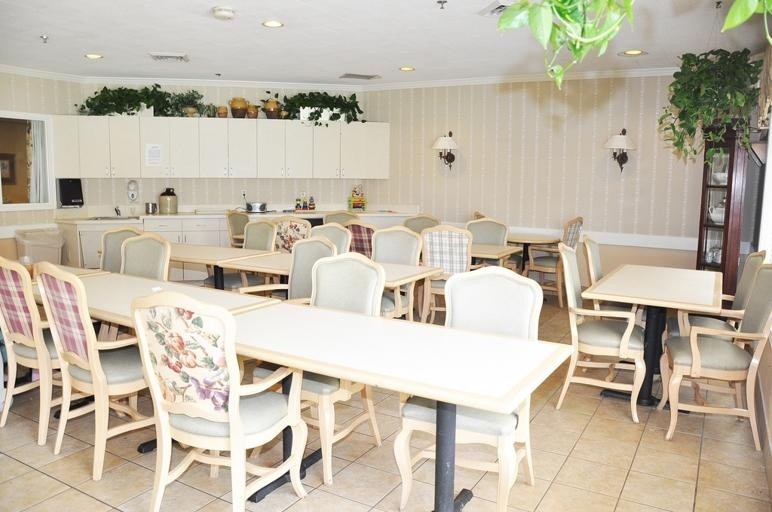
[264,98,282,119]
[218,106,228,118]
[228,97,249,118]
[247,104,258,118]
[206,103,217,117]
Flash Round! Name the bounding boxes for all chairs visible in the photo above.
[555,242,646,424]
[393,265,546,512]
[119,232,172,282]
[238,235,338,306]
[323,210,360,226]
[582,239,647,329]
[0,256,126,446]
[420,225,473,324]
[226,210,250,249]
[204,219,277,296]
[34,261,191,482]
[370,225,423,321]
[522,216,584,308]
[273,216,312,253]
[662,250,768,389]
[131,290,308,512]
[466,217,509,266]
[656,264,772,453]
[99,227,143,273]
[248,252,386,486]
[403,215,440,319]
[344,222,377,259]
[308,221,352,255]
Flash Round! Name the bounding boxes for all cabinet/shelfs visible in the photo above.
[54,204,421,282]
[695,117,752,322]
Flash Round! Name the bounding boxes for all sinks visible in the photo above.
[89,217,139,220]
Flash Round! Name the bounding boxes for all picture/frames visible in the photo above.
[0,153,17,185]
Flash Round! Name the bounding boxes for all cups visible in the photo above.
[145,202,158,215]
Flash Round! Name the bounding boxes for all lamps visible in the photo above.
[604,128,637,172]
[432,131,457,171]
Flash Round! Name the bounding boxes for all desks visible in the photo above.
[217,250,444,322]
[580,263,723,414]
[507,233,560,302]
[231,297,575,512]
[170,242,280,291]
[53,271,283,454]
[470,244,524,274]
[32,263,97,306]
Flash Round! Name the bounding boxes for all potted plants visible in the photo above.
[260,91,366,127]
[170,90,206,117]
[657,47,764,167]
[74,83,174,116]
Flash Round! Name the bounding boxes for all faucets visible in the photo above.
[115,207,121,217]
